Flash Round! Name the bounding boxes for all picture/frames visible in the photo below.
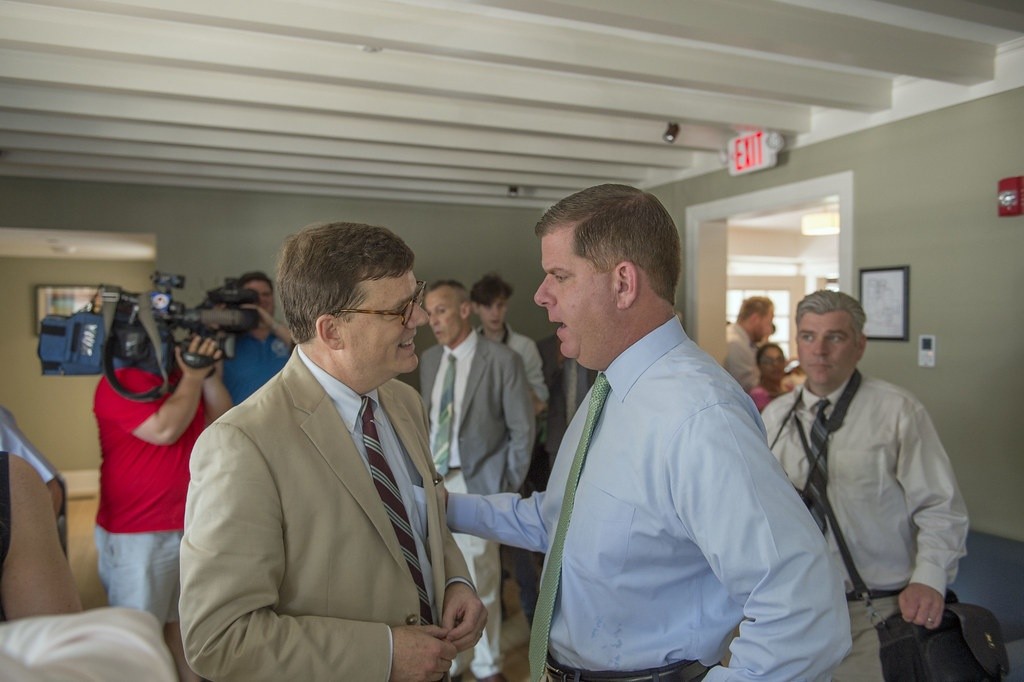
[35,285,98,340]
[857,266,910,341]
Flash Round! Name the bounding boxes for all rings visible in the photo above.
[928,618,934,624]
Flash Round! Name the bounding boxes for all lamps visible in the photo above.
[508,185,517,196]
[718,130,783,175]
[800,213,840,236]
[663,123,680,142]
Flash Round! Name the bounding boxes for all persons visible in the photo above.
[179,223,489,682]
[94,336,233,682]
[469,274,550,630]
[443,184,853,682]
[186,270,296,409]
[0,407,63,524]
[761,289,967,682]
[421,279,536,682]
[722,297,805,413]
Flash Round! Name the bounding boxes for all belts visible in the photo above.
[846,587,903,600]
[544,651,707,682]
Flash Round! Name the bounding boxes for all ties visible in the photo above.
[529,373,610,682]
[431,354,454,476]
[355,392,433,628]
[802,399,830,536]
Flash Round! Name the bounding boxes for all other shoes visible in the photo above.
[477,673,508,682]
[451,674,462,682]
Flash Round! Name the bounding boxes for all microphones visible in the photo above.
[208,288,260,305]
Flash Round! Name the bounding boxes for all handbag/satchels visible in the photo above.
[875,603,1009,682]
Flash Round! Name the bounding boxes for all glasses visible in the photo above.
[322,278,428,326]
